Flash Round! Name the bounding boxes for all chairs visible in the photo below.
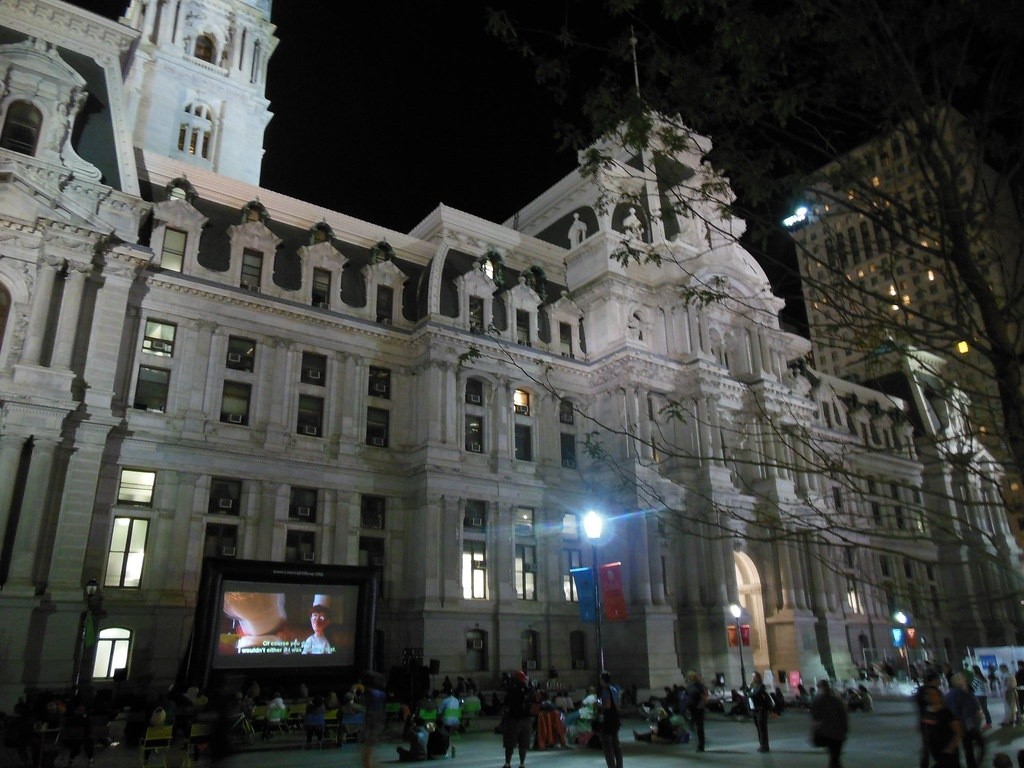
[383,703,403,735]
[418,693,483,741]
[321,709,339,749]
[341,711,366,743]
[139,701,310,768]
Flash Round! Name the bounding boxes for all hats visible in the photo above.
[512,670,526,684]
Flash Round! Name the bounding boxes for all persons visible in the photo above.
[13,675,477,761]
[491,657,1024,768]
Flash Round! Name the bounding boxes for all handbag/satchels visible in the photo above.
[813,723,829,747]
[241,716,255,745]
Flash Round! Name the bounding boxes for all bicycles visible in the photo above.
[227,712,256,746]
[700,677,725,698]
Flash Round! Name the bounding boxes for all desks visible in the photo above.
[19,722,63,768]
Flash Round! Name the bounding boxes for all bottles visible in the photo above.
[452,746,456,757]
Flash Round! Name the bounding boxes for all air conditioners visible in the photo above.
[521,341,532,348]
[563,460,576,468]
[561,414,572,423]
[468,443,481,452]
[524,660,537,670]
[526,564,537,572]
[573,660,585,669]
[517,405,528,412]
[474,560,485,570]
[152,341,167,352]
[472,640,482,649]
[468,394,480,403]
[471,518,483,527]
[220,283,388,565]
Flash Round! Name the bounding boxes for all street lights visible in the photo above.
[74,577,98,697]
[894,611,912,681]
[732,603,748,690]
[583,510,603,697]
